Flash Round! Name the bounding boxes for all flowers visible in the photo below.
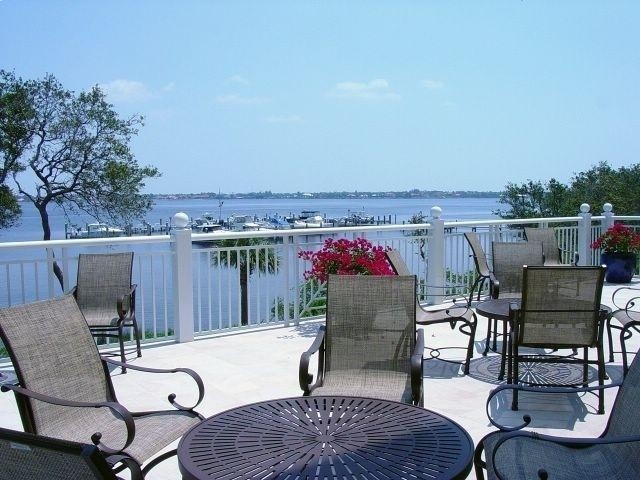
[298,237,396,285]
[589,221,638,255]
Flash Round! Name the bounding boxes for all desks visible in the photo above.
[476,298,615,381]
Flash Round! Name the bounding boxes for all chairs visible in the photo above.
[489,241,544,352]
[388,251,480,375]
[474,349,640,480]
[68,251,143,376]
[604,284,640,379]
[524,228,580,296]
[464,229,493,305]
[506,262,608,413]
[299,274,424,424]
[0,426,118,480]
[0,294,205,480]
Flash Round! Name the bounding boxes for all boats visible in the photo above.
[79,224,123,237]
[337,209,377,226]
[195,212,259,231]
[286,210,333,229]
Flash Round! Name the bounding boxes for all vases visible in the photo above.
[602,252,636,282]
